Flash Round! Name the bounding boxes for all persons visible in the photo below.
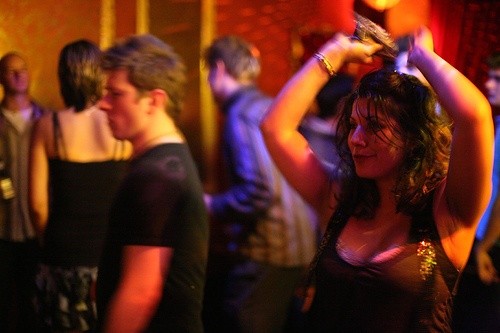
[471,48,499,332]
[259,26,500,333]
[99,33,210,333]
[29,35,134,332]
[201,34,317,333]
[0,51,48,333]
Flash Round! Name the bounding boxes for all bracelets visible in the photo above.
[314,53,334,78]
